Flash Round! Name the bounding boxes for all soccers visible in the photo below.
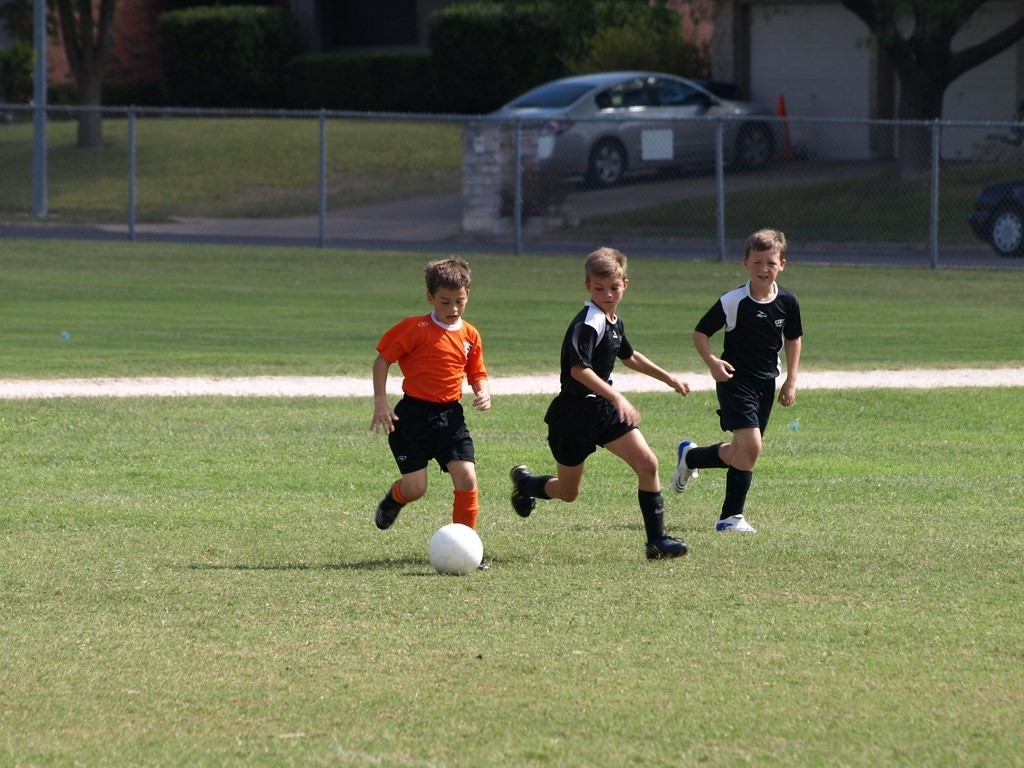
[429,523,483,576]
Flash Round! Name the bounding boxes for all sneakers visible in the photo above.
[375,483,406,530]
[674,441,698,494]
[645,536,686,560]
[510,465,535,518]
[716,515,756,533]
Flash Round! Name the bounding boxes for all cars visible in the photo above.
[645,81,751,108]
[465,70,777,194]
[967,178,1024,257]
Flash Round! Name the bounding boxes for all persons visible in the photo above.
[368,256,492,571]
[510,248,690,560]
[672,229,804,531]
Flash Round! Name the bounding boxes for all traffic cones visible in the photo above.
[771,91,799,158]
